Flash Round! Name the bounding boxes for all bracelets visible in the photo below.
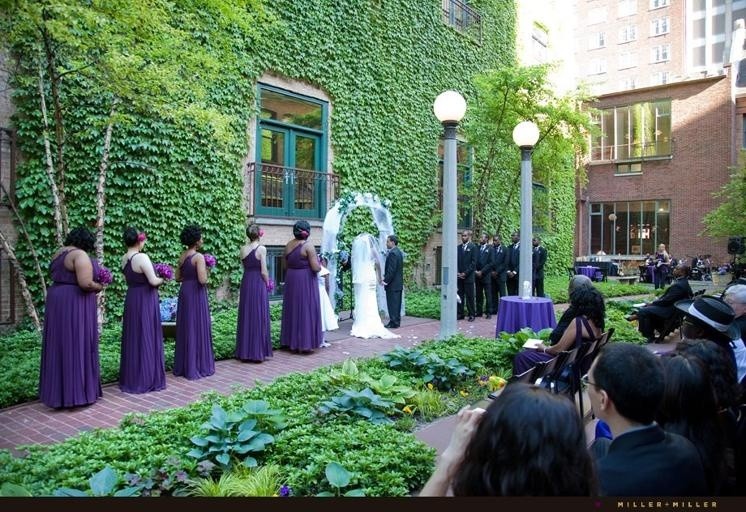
[378,279,383,285]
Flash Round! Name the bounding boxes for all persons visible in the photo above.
[319,256,331,270]
[119,227,164,395]
[475,232,496,322]
[280,220,322,354]
[532,237,547,298]
[492,235,510,315]
[720,283,746,339]
[236,224,275,363]
[643,242,676,290]
[313,254,339,348]
[383,235,403,330]
[548,274,594,344]
[350,233,387,339]
[687,340,743,449]
[506,231,520,297]
[173,226,215,381]
[488,288,606,401]
[676,255,686,267]
[418,382,598,499]
[672,294,736,389]
[456,230,476,322]
[624,263,690,343]
[689,254,733,279]
[38,225,112,409]
[580,344,706,506]
[657,350,714,448]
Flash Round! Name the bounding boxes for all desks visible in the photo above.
[495,295,556,339]
[574,264,601,281]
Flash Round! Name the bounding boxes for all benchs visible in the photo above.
[615,276,640,284]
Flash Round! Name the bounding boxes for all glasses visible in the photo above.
[581,375,601,388]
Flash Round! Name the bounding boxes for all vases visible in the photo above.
[712,273,732,286]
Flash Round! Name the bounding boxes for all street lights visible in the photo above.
[609,213,617,255]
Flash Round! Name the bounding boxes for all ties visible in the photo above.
[480,245,484,255]
[462,245,465,256]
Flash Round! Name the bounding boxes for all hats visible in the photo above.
[674,294,741,340]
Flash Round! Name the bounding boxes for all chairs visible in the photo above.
[468,362,542,411]
[568,267,576,279]
[554,342,586,402]
[489,348,565,399]
[592,268,608,283]
[577,328,615,422]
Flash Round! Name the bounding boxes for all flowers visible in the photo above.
[204,253,216,265]
[97,268,113,284]
[718,266,728,274]
[267,279,273,291]
[154,260,172,279]
